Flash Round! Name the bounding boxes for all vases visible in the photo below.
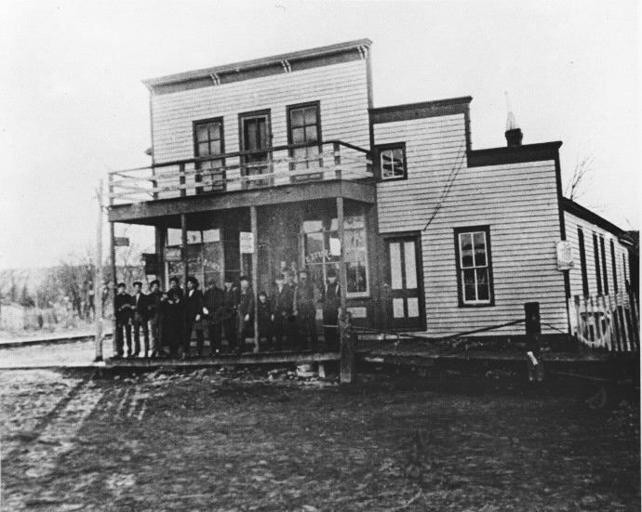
[505,129,523,146]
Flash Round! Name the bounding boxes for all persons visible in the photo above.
[112,267,340,360]
